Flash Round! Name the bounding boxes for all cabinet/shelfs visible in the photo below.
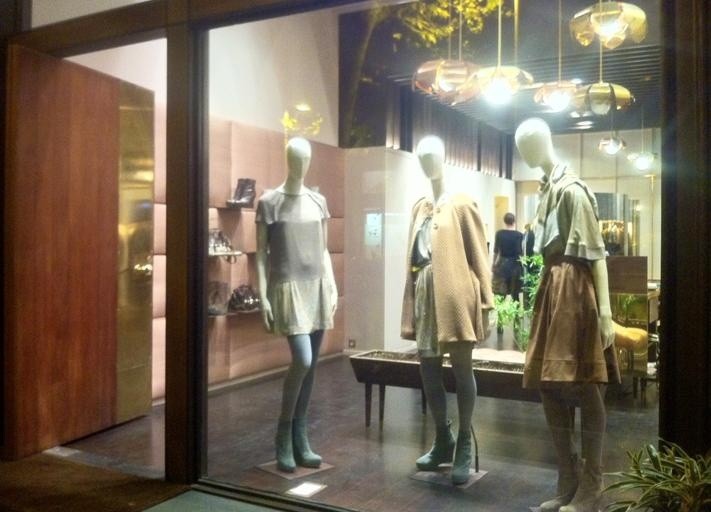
[152,105,346,398]
[346,150,519,358]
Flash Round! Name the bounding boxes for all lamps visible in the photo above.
[626,115,658,172]
[411,1,481,105]
[535,3,586,112]
[569,0,647,49]
[467,1,534,105]
[598,115,627,156]
[573,0,632,115]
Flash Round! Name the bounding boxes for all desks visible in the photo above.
[610,291,660,363]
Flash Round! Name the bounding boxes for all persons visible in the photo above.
[254,136,340,473]
[400,134,495,484]
[515,117,621,512]
[492,211,527,334]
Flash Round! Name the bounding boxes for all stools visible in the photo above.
[613,322,649,409]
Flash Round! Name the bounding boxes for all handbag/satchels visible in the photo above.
[228,280,260,312]
[207,227,237,265]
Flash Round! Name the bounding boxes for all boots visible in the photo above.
[225,178,246,206]
[208,281,230,316]
[451,428,474,485]
[556,464,608,512]
[416,421,457,472]
[205,281,218,306]
[272,420,299,474]
[292,418,323,468]
[236,179,257,208]
[538,454,582,512]
